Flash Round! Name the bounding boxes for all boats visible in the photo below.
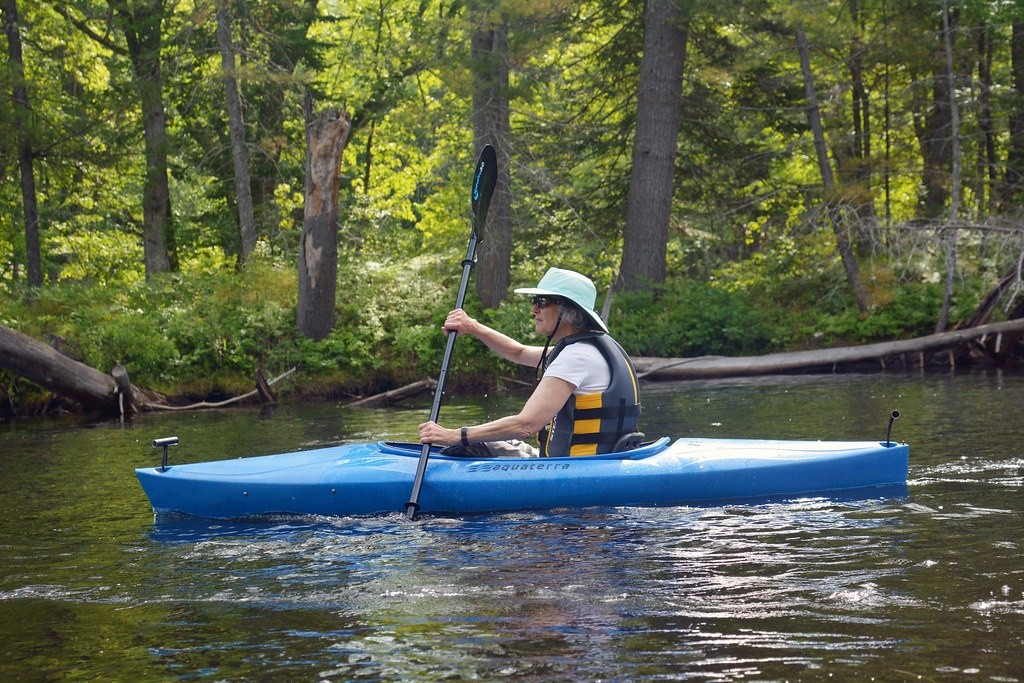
[136,440,909,523]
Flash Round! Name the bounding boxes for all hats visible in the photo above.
[514,267,609,333]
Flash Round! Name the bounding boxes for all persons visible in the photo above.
[416,266,640,457]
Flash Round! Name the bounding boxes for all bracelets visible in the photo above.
[460,426,472,446]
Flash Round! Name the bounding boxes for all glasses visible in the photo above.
[530,296,562,308]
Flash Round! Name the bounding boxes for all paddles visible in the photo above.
[406,143,500,520]
